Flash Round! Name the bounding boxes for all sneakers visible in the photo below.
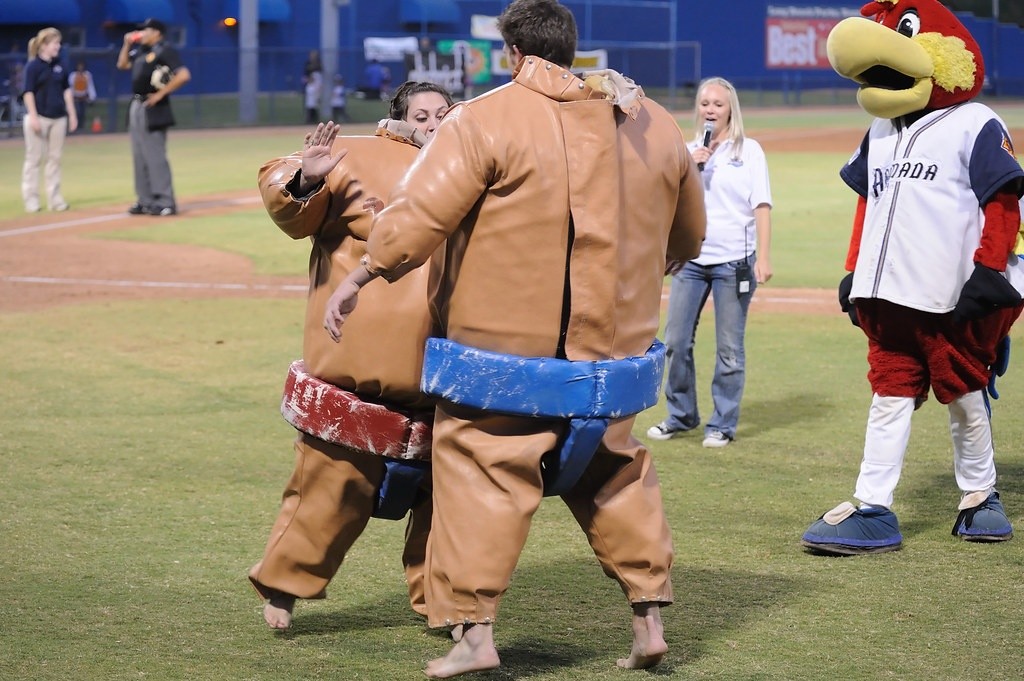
[701,429,729,448]
[647,422,679,439]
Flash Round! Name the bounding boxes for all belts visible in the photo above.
[132,93,143,100]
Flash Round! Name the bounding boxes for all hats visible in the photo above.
[138,17,166,34]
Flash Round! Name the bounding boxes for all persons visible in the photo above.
[300,49,324,124]
[646,76,773,448]
[322,0,708,679]
[247,81,465,630]
[16,27,79,214]
[354,59,389,99]
[68,57,98,130]
[407,34,442,71]
[330,74,352,124]
[115,17,192,217]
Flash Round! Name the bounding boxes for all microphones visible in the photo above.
[697,121,715,171]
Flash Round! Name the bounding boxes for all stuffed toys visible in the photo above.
[800,0,1024,556]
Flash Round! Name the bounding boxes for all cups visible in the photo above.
[128,31,143,44]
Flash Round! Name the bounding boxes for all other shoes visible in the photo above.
[150,207,175,215]
[128,204,151,213]
[49,202,69,211]
[27,204,41,213]
